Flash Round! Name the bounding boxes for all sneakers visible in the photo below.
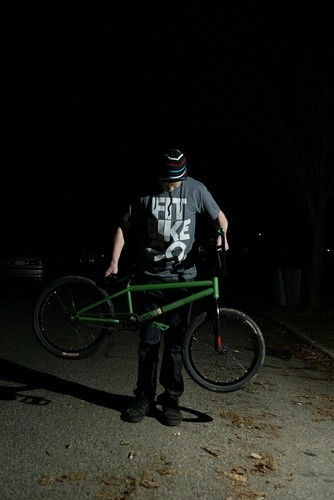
[121,398,156,423]
[160,399,183,426]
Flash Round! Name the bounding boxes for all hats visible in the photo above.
[157,149,188,183]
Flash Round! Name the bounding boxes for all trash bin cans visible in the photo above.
[273,268,302,308]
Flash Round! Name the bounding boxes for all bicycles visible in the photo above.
[33,228,265,393]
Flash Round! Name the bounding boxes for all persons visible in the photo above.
[105,148,229,426]
